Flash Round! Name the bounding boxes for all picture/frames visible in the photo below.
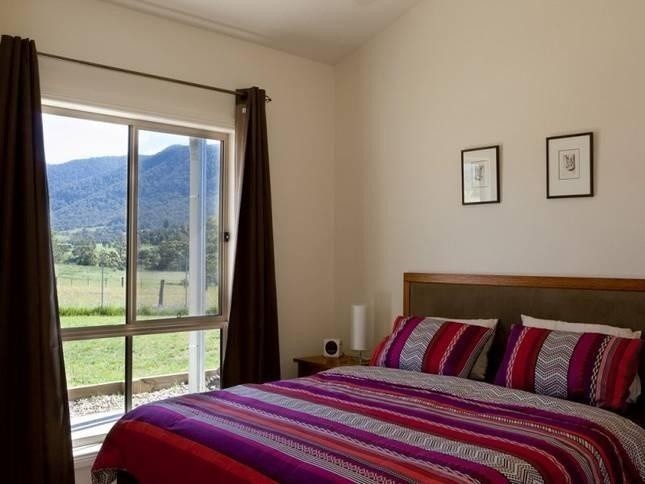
[460,144,501,206]
[545,130,595,200]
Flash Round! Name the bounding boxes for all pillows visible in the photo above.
[368,313,645,412]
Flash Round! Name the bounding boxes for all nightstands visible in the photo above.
[292,354,360,377]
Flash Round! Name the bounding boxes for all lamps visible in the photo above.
[350,303,372,365]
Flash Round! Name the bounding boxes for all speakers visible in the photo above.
[324,337,343,358]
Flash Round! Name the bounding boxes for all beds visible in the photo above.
[88,271,645,483]
